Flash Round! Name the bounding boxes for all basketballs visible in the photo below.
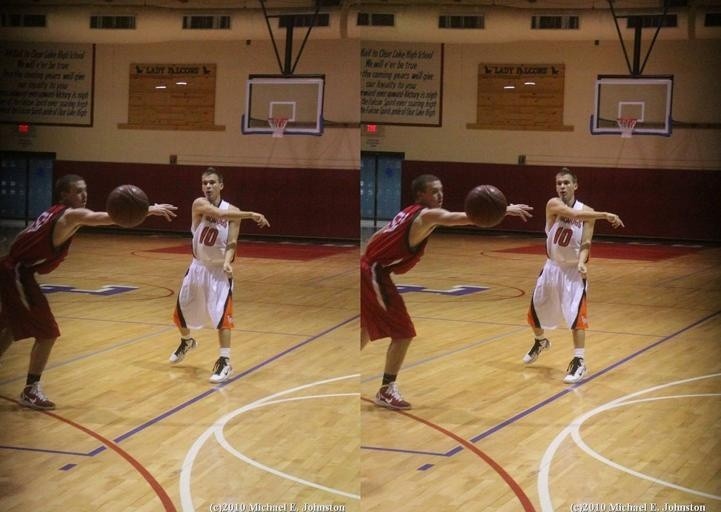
[107,185,149,227]
[465,185,507,228]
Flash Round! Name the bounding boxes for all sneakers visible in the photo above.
[169,337,198,364]
[562,355,586,384]
[522,337,551,364]
[375,381,410,410]
[209,356,231,383]
[18,381,56,410]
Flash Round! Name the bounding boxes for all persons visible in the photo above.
[169,167,271,383]
[360,174,534,410]
[1,174,179,411]
[523,166,624,384]
